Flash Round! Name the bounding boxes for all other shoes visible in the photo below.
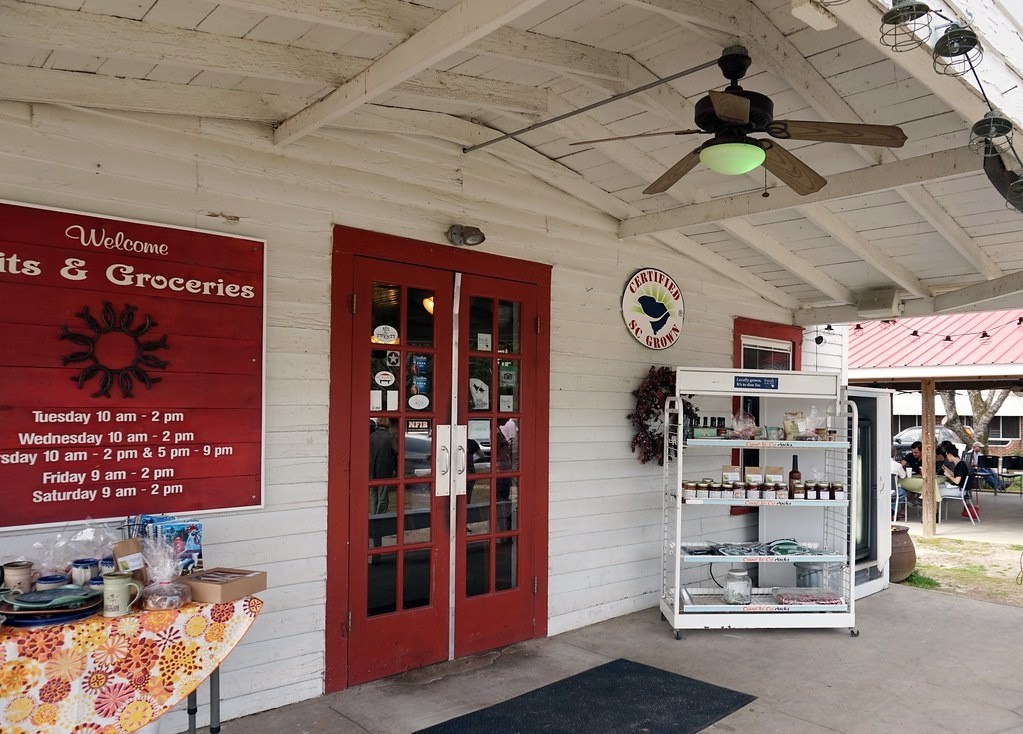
[999,483,1009,489]
[466,528,471,532]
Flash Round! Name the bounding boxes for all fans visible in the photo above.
[567,44,908,196]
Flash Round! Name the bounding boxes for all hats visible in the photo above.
[972,442,985,447]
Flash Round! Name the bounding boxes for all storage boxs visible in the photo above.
[177,566,268,605]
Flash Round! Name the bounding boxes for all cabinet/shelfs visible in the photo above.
[658,366,860,638]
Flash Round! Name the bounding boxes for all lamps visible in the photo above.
[697,130,766,176]
[445,224,485,246]
[423,296,434,316]
[1005,174,1023,214]
[813,316,1023,347]
[932,24,985,78]
[879,0,933,54]
[967,109,1014,158]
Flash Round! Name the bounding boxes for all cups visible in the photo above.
[72,558,99,585]
[102,571,141,618]
[36,575,68,590]
[906,468,912,477]
[768,427,783,439]
[101,558,115,577]
[89,577,103,591]
[3,560,40,594]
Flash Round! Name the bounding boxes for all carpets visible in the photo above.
[413,659,762,734]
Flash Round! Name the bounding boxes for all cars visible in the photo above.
[389,430,494,493]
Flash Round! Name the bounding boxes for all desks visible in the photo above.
[0,596,264,734]
[898,474,948,524]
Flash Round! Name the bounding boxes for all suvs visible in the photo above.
[890,424,979,476]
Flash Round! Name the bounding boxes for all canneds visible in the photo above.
[682,478,844,499]
[815,428,837,441]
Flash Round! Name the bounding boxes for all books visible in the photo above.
[120,515,203,576]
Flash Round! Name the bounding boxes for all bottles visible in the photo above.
[789,455,801,499]
[684,414,725,437]
[724,570,752,604]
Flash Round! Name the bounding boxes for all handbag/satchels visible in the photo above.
[962,504,979,519]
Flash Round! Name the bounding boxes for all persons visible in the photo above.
[497,427,514,548]
[466,439,485,533]
[965,442,1012,490]
[900,441,922,507]
[936,438,971,524]
[369,418,398,515]
[891,446,908,521]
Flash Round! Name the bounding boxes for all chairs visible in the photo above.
[962,450,1023,496]
[938,473,982,527]
[891,473,908,523]
